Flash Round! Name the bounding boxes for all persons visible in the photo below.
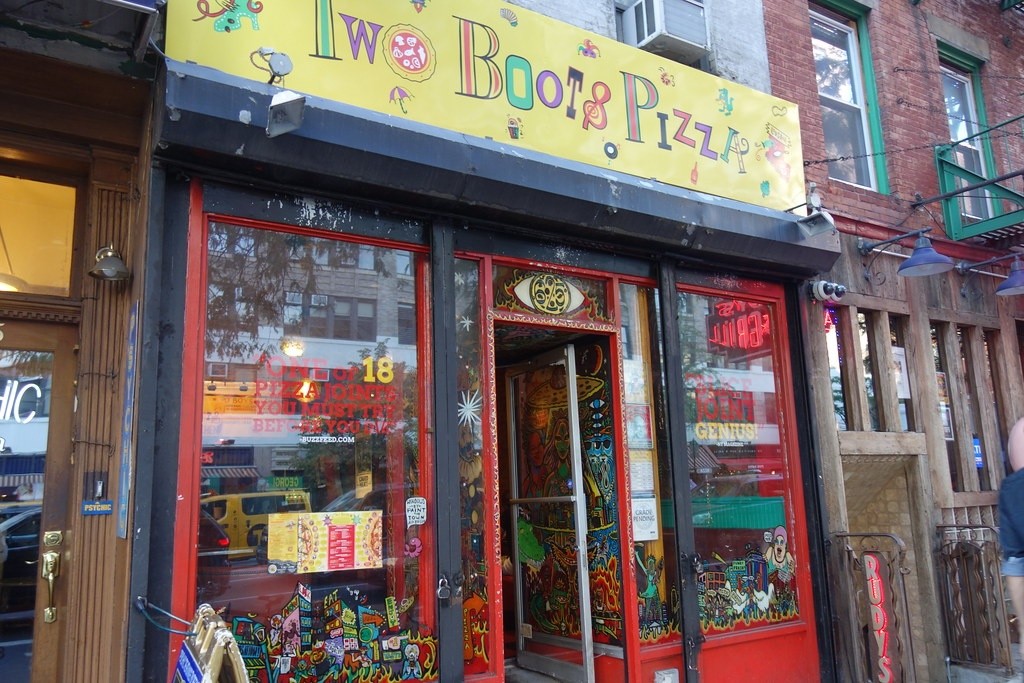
[999,415,1024,660]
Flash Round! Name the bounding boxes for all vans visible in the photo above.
[198,492,314,565]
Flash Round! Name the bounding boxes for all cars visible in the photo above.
[0,494,233,634]
[688,470,787,500]
[318,482,422,512]
